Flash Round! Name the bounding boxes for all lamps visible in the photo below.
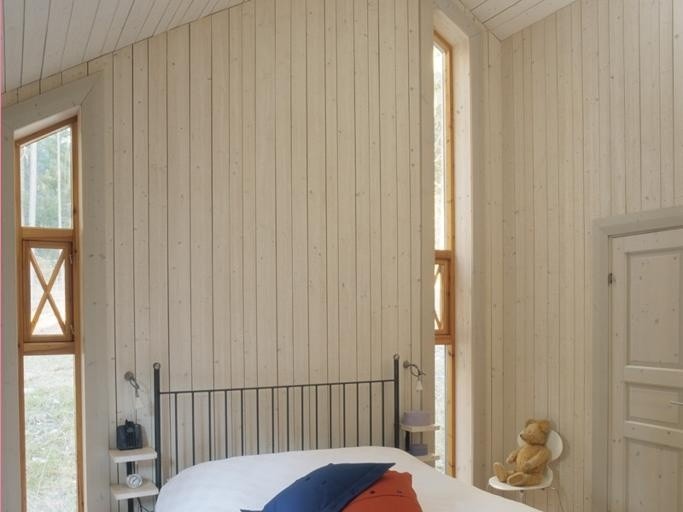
[124,372,144,409]
[403,361,425,391]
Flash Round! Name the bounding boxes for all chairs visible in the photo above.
[488,429,563,503]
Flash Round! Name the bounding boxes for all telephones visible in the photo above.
[117,419,143,450]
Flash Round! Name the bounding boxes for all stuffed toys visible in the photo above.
[494,419,551,485]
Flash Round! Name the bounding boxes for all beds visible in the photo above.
[154,354,545,512]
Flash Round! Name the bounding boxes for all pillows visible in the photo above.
[240,463,422,512]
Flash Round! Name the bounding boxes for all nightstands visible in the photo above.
[110,447,159,512]
[400,423,439,468]
